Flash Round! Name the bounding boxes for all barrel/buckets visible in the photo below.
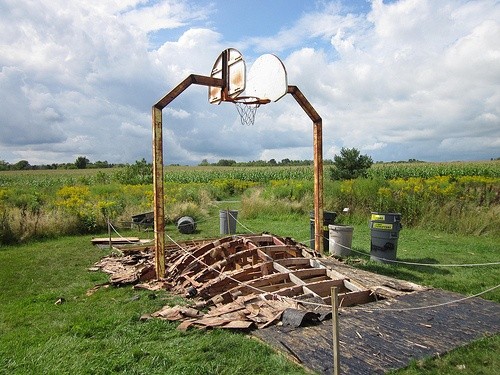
[219,210,237,234]
[369,210,403,264]
[176,216,196,234]
[309,217,336,253]
[327,223,354,258]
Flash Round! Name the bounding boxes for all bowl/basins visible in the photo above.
[310,210,337,221]
